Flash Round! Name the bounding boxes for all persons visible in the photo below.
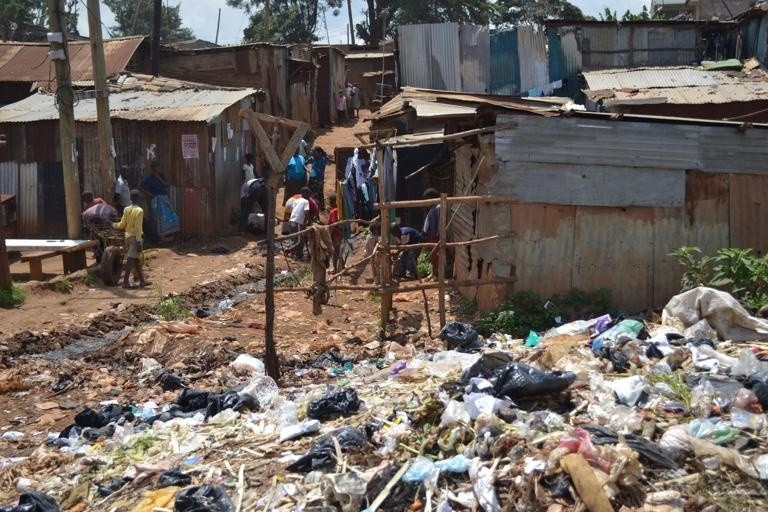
[345,83,352,113]
[138,160,180,242]
[350,83,361,119]
[94,190,121,232]
[111,165,133,237]
[421,187,448,282]
[106,188,154,288]
[388,221,424,279]
[239,137,351,276]
[335,90,348,128]
[351,200,394,285]
[82,189,107,259]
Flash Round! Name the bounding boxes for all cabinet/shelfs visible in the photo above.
[0,194,20,264]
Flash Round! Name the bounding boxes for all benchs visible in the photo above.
[20,249,58,276]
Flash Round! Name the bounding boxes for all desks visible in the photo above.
[3,238,97,274]
